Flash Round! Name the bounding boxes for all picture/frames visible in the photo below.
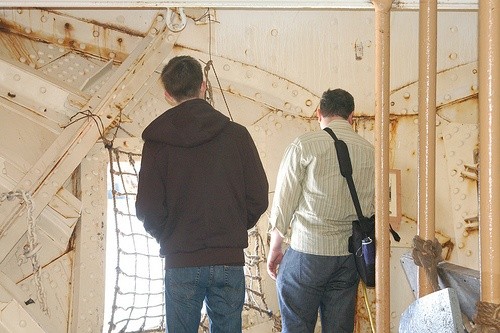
[388,169,401,224]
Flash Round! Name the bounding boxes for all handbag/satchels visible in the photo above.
[348,216,377,289]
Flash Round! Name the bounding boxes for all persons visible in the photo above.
[266,88,377,333]
[135,55,269,333]
